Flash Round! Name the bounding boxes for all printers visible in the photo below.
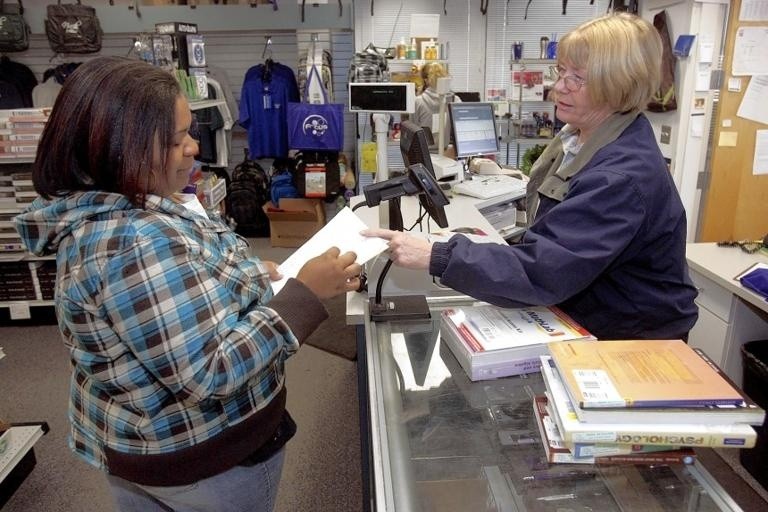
[427,154,464,188]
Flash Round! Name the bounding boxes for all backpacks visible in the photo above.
[230,160,271,239]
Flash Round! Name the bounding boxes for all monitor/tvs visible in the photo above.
[399,120,449,229]
[448,101,501,160]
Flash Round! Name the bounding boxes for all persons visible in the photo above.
[358,10,698,344]
[13,56,365,511]
[408,64,464,149]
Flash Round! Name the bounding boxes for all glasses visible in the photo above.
[547,64,589,94]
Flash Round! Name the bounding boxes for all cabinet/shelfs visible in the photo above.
[503,61,566,177]
[344,162,767,511]
[0,58,229,328]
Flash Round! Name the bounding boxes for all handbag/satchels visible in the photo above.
[46,4,103,54]
[285,101,343,151]
[0,4,30,51]
[294,153,340,201]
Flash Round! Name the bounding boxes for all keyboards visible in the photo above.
[439,183,454,198]
[452,175,528,200]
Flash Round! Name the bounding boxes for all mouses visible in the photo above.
[507,172,523,180]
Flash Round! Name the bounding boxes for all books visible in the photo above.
[439,303,600,383]
[532,337,766,464]
